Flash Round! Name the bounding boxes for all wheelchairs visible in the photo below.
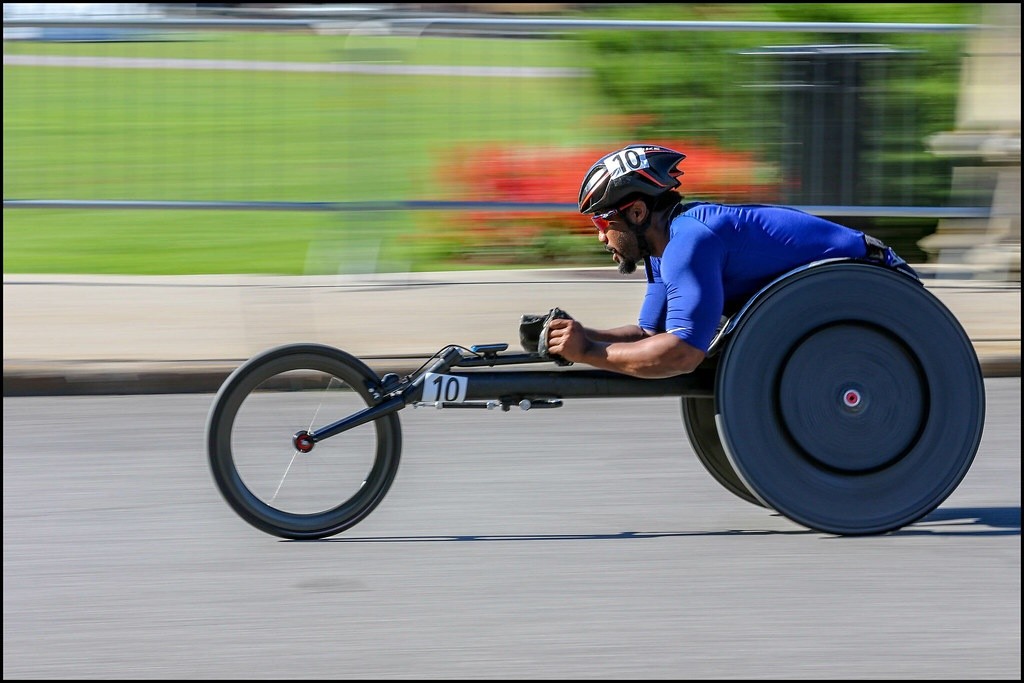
[204,256,987,541]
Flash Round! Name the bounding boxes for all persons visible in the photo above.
[518,145,924,379]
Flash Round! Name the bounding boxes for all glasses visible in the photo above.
[590,199,639,231]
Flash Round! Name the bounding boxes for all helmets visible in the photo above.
[578,144,687,215]
